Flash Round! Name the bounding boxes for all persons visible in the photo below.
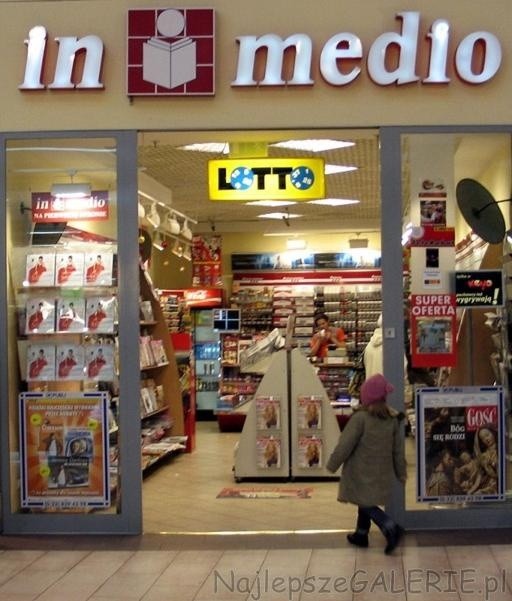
[28,255,48,284]
[426,201,443,223]
[304,402,320,429]
[29,302,43,331]
[327,373,408,555]
[30,349,48,379]
[262,439,279,468]
[88,348,106,377]
[429,407,453,462]
[309,313,346,357]
[468,428,498,496]
[88,302,107,330]
[305,440,321,468]
[426,448,456,497]
[262,402,278,430]
[86,255,105,282]
[58,256,76,283]
[42,432,63,456]
[59,349,77,377]
[59,302,76,331]
[456,452,478,493]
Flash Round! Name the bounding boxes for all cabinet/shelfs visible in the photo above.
[291,348,345,481]
[15,247,184,514]
[218,350,352,431]
[423,235,503,395]
[234,347,289,482]
[157,288,195,455]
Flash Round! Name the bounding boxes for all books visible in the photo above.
[255,396,282,431]
[56,296,85,333]
[138,291,157,323]
[108,407,188,479]
[26,343,55,382]
[85,252,113,286]
[55,251,85,287]
[86,296,114,333]
[24,252,55,288]
[85,324,169,380]
[299,433,324,469]
[56,344,85,381]
[81,364,178,433]
[25,296,55,335]
[297,395,325,433]
[86,343,115,381]
[257,432,284,469]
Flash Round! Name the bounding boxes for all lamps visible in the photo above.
[50,182,91,200]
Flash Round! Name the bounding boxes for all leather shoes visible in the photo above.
[348,532,369,547]
[384,525,404,555]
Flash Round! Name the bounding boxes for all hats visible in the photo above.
[361,374,394,406]
